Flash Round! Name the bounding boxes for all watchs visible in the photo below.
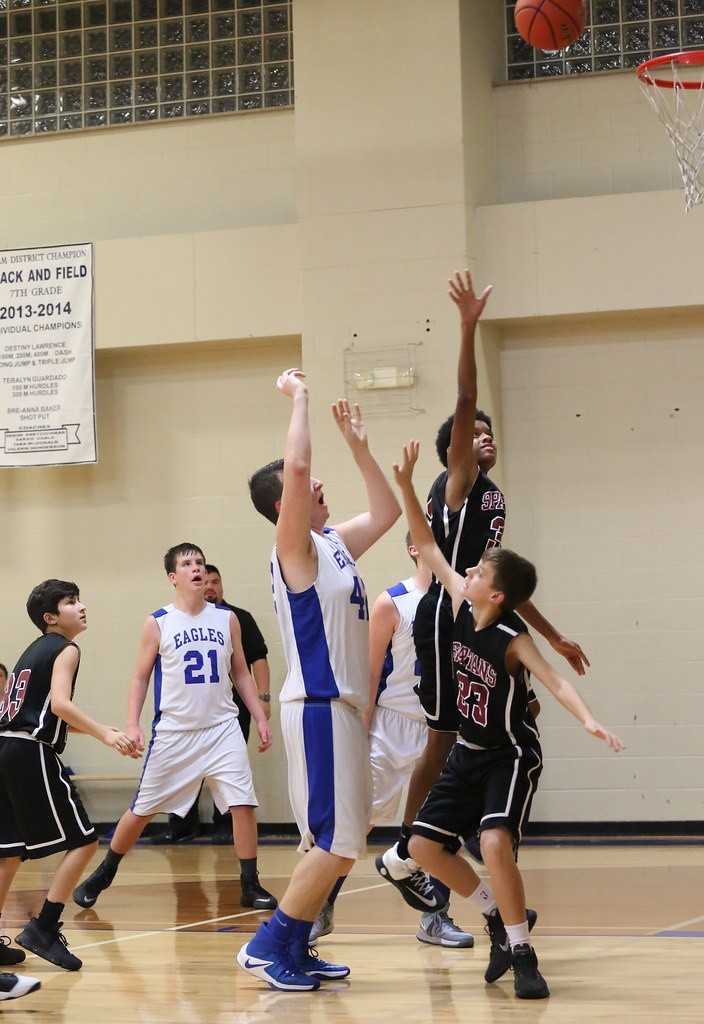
[259,693,271,701]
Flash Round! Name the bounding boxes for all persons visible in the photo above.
[72,540,279,910]
[1,578,138,1000]
[374,272,590,913]
[309,524,476,946]
[149,564,273,843]
[237,366,402,992]
[392,440,630,1000]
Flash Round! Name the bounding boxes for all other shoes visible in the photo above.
[212,822,234,845]
[171,824,196,842]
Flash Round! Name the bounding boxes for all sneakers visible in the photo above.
[318,981,350,1003]
[74,910,102,932]
[508,943,550,999]
[73,860,118,908]
[14,916,83,971]
[417,901,474,946]
[239,991,316,1024]
[481,907,537,983]
[288,938,350,978]
[484,982,549,1023]
[34,970,81,1009]
[415,944,475,973]
[240,871,279,909]
[0,972,41,1001]
[375,842,448,912]
[308,900,334,945]
[236,921,321,991]
[0,936,26,966]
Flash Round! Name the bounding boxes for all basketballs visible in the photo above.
[513,1,589,53]
